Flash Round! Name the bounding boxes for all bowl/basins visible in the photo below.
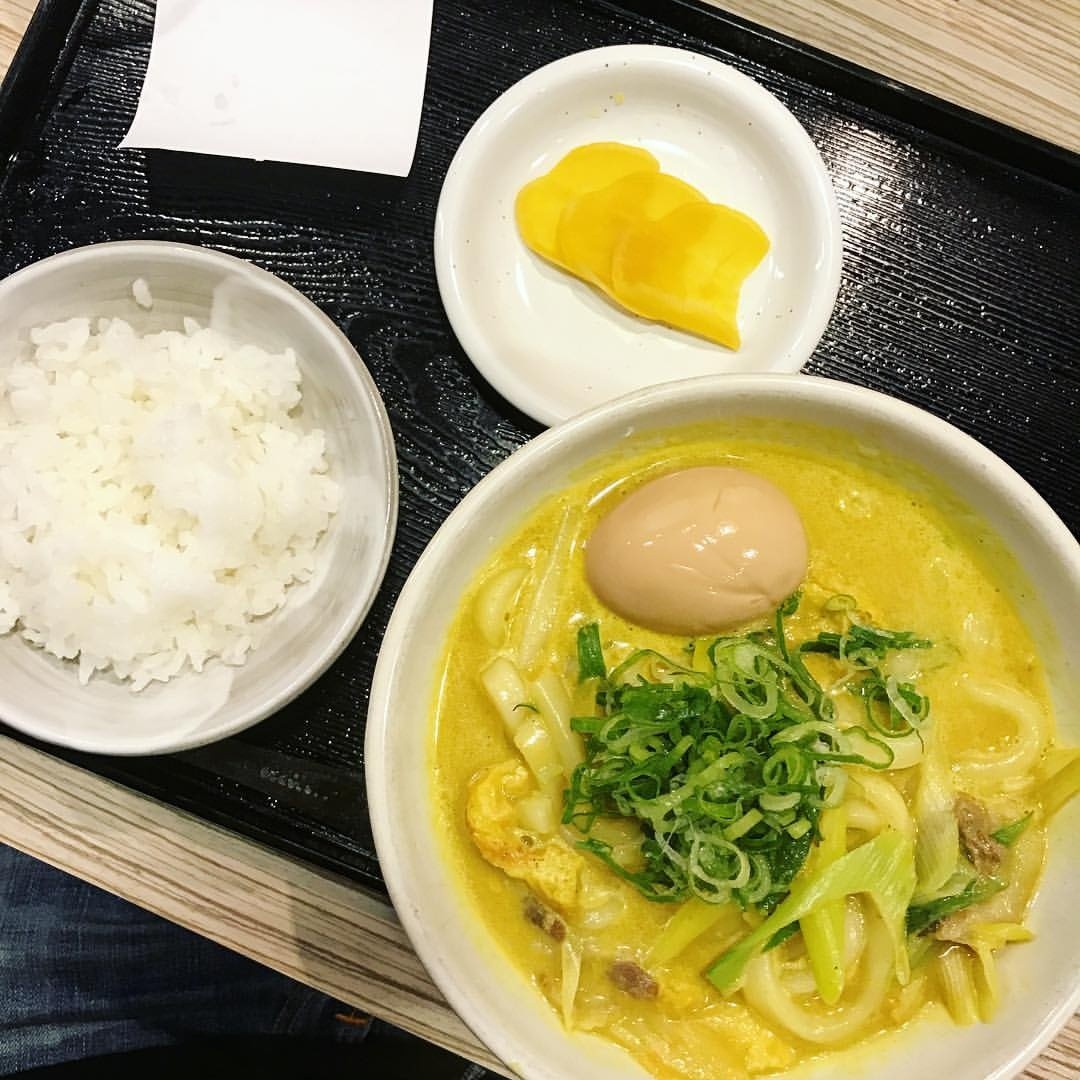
[365,373,1079,1078]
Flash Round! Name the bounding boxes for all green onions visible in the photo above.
[560,595,934,906]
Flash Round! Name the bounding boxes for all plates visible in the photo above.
[435,47,846,422]
[0,241,402,756]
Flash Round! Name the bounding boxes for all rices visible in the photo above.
[0,279,342,693]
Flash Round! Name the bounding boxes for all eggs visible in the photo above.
[588,464,810,634]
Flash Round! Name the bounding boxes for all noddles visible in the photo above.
[473,518,1044,1078]
